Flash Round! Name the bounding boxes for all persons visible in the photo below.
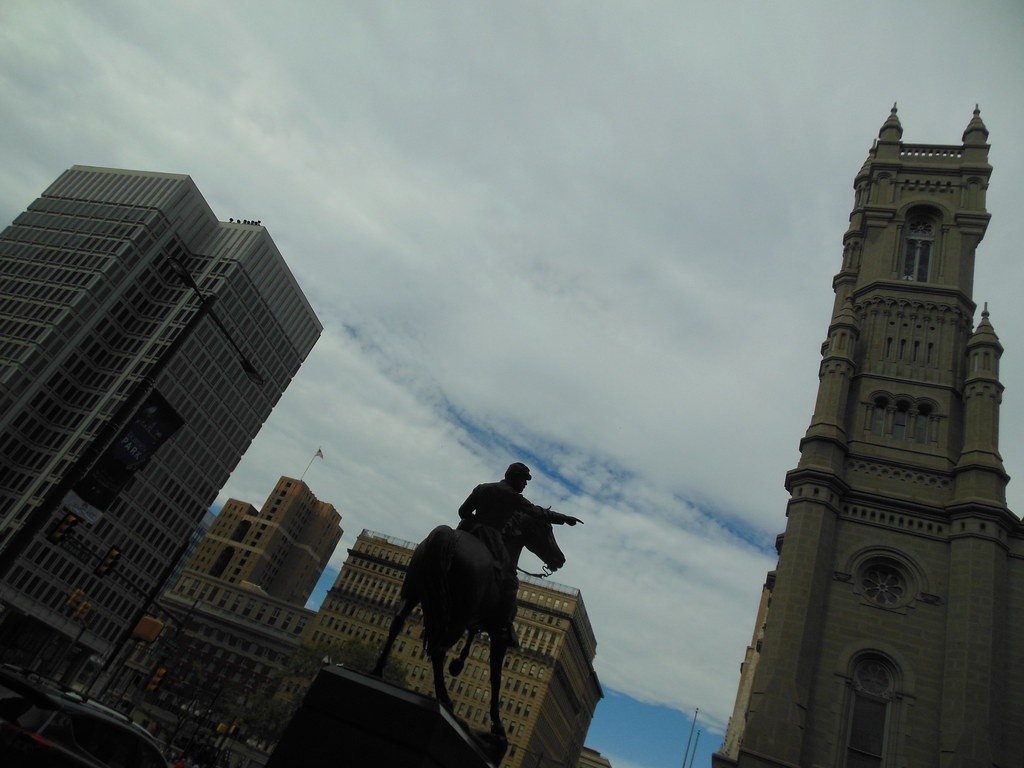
[458,461,585,640]
[153,738,192,768]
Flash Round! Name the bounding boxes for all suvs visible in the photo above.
[0,661,172,768]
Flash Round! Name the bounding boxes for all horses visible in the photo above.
[369,504,568,758]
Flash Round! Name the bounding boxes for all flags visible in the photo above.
[315,449,325,459]
